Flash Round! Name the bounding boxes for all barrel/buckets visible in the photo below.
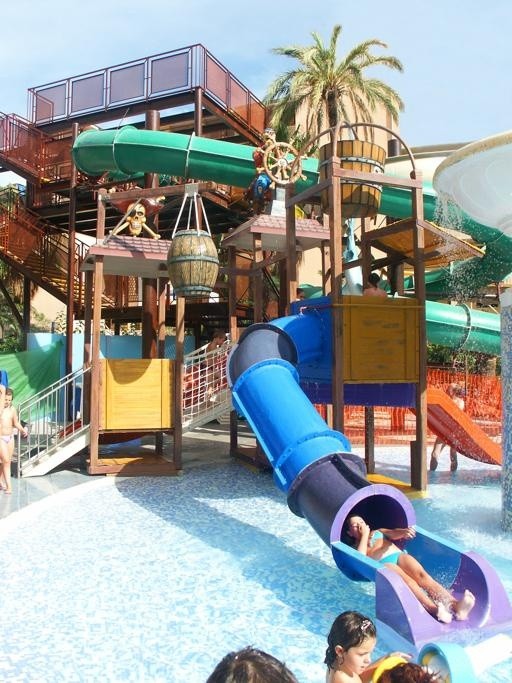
[169,227,219,297]
[317,140,386,218]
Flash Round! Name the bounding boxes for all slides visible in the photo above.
[73,124,512,359]
[407,382,503,467]
[226,309,512,651]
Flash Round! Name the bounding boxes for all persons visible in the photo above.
[296,287,306,301]
[345,513,476,624]
[321,609,413,682]
[0,383,29,494]
[206,645,300,683]
[201,327,228,398]
[0,383,7,415]
[363,272,387,296]
[429,382,465,471]
